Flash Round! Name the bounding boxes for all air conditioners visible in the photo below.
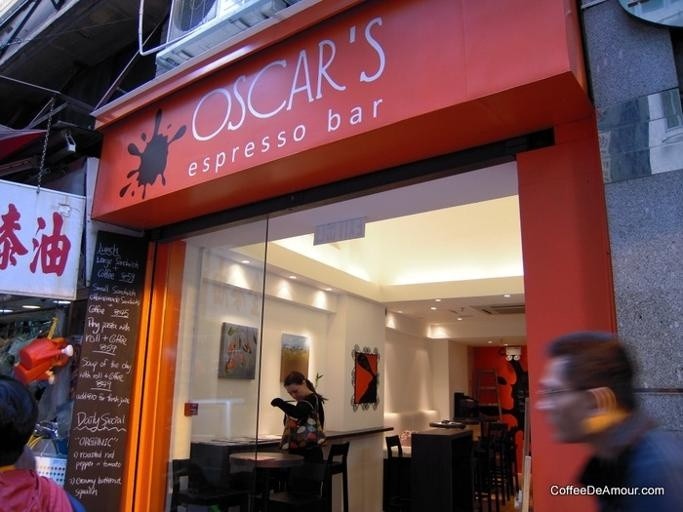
[156,0,299,70]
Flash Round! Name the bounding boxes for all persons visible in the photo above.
[0,372,88,511]
[270,369,328,510]
[533,328,682,510]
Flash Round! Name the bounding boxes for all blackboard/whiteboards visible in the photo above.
[64,230,148,512]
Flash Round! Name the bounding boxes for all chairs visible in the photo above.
[386,435,411,512]
[474,420,519,512]
[171,460,248,512]
[323,441,349,512]
[255,460,331,512]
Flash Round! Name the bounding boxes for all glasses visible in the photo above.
[535,386,593,402]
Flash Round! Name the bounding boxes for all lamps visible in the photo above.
[506,346,521,361]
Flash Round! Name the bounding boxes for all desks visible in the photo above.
[411,429,473,512]
[188,418,394,505]
[229,452,304,512]
[429,420,466,428]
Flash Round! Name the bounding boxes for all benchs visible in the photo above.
[383,410,441,458]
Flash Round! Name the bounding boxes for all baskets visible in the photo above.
[32,451,68,486]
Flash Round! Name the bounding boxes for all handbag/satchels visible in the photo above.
[280,410,327,450]
